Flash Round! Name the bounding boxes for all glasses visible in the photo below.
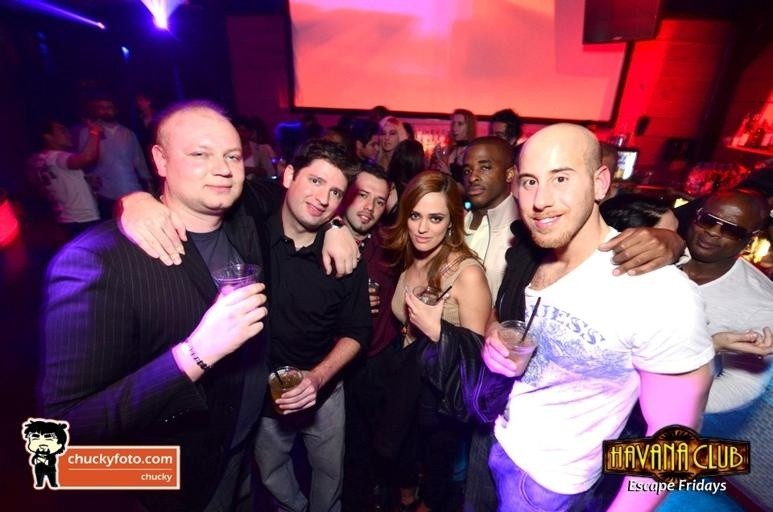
[697,206,760,241]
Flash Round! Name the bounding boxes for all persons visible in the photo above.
[480,124,714,512]
[115,136,374,508]
[455,138,691,311]
[597,186,773,360]
[673,190,773,452]
[392,168,491,511]
[339,160,401,509]
[23,88,771,242]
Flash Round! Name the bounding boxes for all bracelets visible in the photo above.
[40,101,268,512]
[177,335,217,376]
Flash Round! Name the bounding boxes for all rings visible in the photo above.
[357,254,363,260]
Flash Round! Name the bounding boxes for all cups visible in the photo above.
[209,263,262,295]
[411,286,446,305]
[489,320,539,378]
[267,366,302,415]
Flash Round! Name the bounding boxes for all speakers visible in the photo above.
[582,0,664,41]
